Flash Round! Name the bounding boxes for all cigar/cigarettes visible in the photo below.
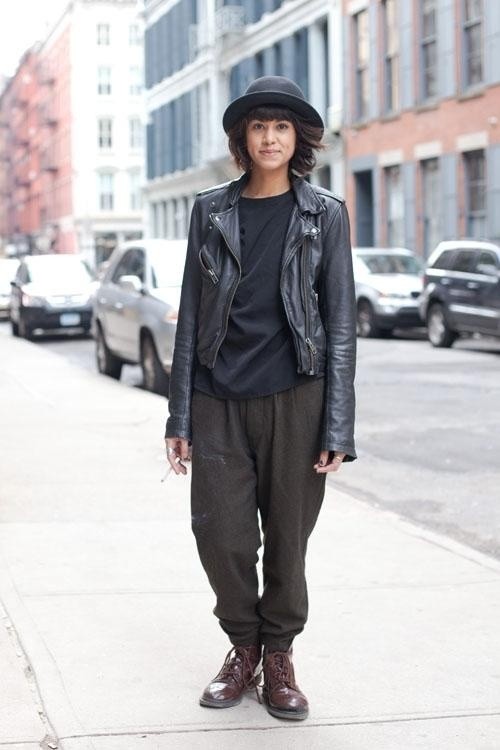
[161,457,181,483]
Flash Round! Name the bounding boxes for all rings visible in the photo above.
[167,446,176,458]
[334,456,343,462]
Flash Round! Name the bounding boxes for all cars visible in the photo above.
[351,240,500,347]
[0,239,188,393]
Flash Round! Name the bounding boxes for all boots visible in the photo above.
[260,644,308,723]
[198,642,263,710]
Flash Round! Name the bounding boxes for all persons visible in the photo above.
[166,76,358,721]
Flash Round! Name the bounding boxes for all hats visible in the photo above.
[220,72,324,144]
